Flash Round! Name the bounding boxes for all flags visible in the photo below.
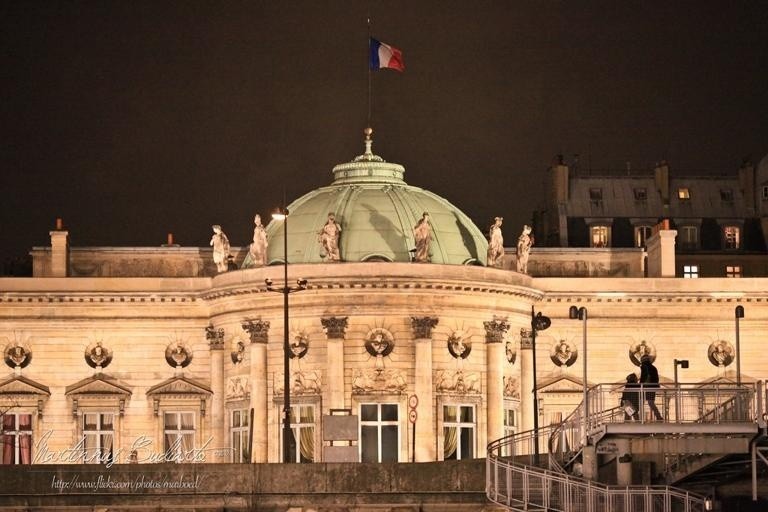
[368,36,406,74]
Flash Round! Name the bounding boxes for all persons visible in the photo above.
[317,212,342,262]
[208,222,231,275]
[620,373,642,423]
[248,212,269,266]
[486,214,505,270]
[638,353,665,423]
[412,213,437,263]
[516,223,534,275]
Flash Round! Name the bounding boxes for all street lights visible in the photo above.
[733,306,746,383]
[530,304,551,469]
[268,181,293,462]
[566,305,588,442]
[673,357,690,422]
